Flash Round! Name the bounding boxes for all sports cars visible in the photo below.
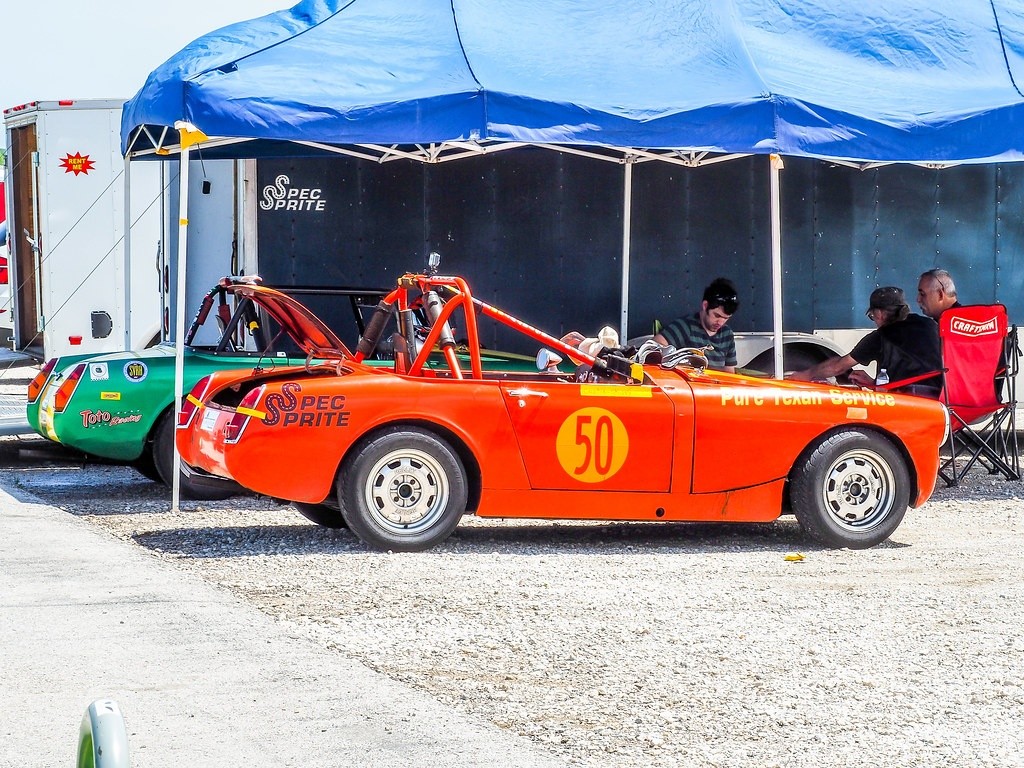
[172,251,952,554]
[26,286,578,500]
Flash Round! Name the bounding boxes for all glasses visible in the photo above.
[710,290,739,303]
[867,312,876,321]
[920,272,945,291]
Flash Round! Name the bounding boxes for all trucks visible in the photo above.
[0,98,255,436]
[731,327,1024,437]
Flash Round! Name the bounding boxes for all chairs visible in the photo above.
[878,305,1021,485]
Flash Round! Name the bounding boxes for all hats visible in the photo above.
[865,287,908,315]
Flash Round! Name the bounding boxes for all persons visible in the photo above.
[652,277,738,377]
[915,269,964,331]
[786,287,943,405]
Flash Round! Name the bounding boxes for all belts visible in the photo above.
[892,386,941,397]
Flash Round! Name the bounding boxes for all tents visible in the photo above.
[122,0,1024,511]
